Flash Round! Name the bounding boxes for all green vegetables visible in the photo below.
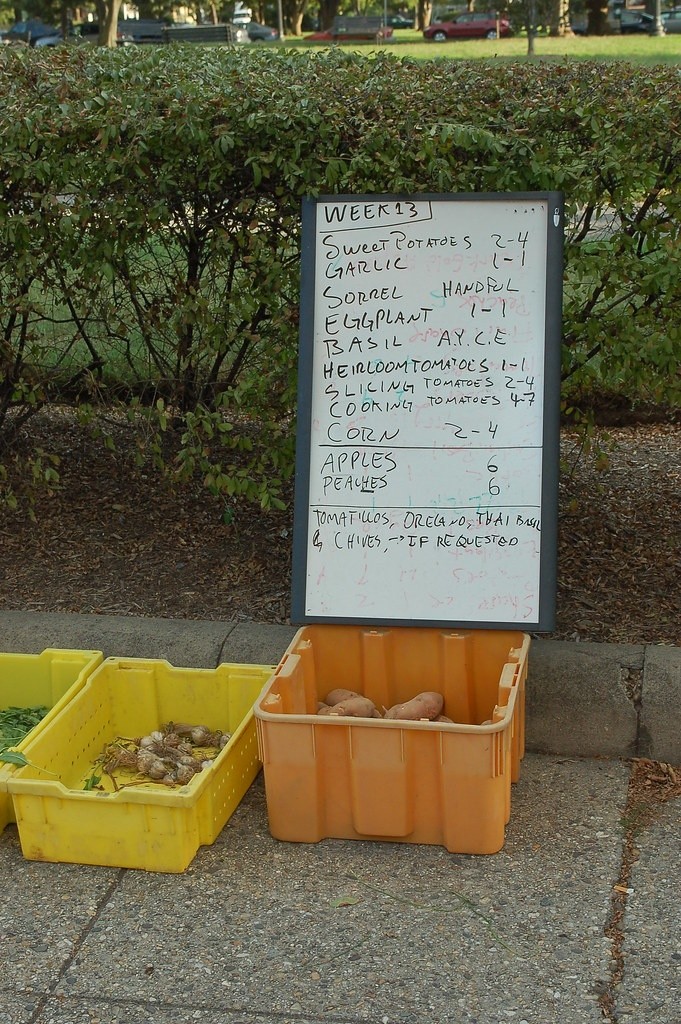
[0,704,102,790]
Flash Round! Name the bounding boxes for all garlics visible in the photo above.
[110,723,235,785]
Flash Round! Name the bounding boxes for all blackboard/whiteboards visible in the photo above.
[291,191,563,632]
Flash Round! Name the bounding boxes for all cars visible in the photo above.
[0,19,58,44]
[661,11,681,33]
[303,16,393,41]
[237,22,279,41]
[389,16,414,29]
[573,10,664,35]
[33,24,124,47]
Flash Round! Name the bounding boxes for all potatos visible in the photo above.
[310,687,493,725]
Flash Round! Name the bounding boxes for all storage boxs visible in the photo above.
[0,657,276,872]
[253,632,531,856]
[0,649,102,834]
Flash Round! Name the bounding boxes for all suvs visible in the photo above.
[423,11,513,40]
